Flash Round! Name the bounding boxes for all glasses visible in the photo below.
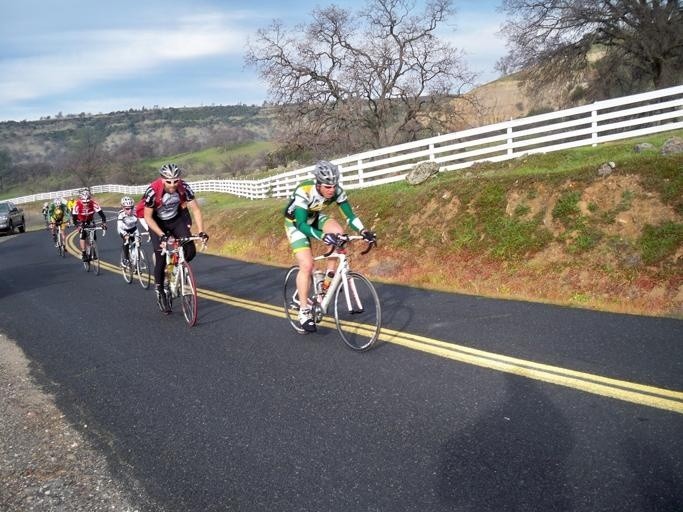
[165,180,178,184]
[83,202,89,204]
[125,208,132,210]
[321,183,336,189]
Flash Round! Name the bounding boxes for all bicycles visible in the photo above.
[280,232,383,353]
[118,231,153,290]
[154,235,209,328]
[42,212,108,277]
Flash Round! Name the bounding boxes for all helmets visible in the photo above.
[315,160,339,185]
[158,163,181,179]
[54,199,62,208]
[77,187,91,200]
[120,196,134,206]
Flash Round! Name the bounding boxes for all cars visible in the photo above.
[0,200,27,235]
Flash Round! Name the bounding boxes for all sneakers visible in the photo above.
[155,292,172,312]
[81,251,88,262]
[296,311,316,332]
[122,259,131,266]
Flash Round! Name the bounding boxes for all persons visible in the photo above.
[39,195,77,249]
[282,160,376,332]
[70,189,107,262]
[142,162,209,316]
[115,196,150,275]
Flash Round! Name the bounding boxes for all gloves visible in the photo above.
[198,231,208,244]
[359,228,376,244]
[101,223,107,230]
[322,233,340,245]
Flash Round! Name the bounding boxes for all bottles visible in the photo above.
[322,270,334,288]
